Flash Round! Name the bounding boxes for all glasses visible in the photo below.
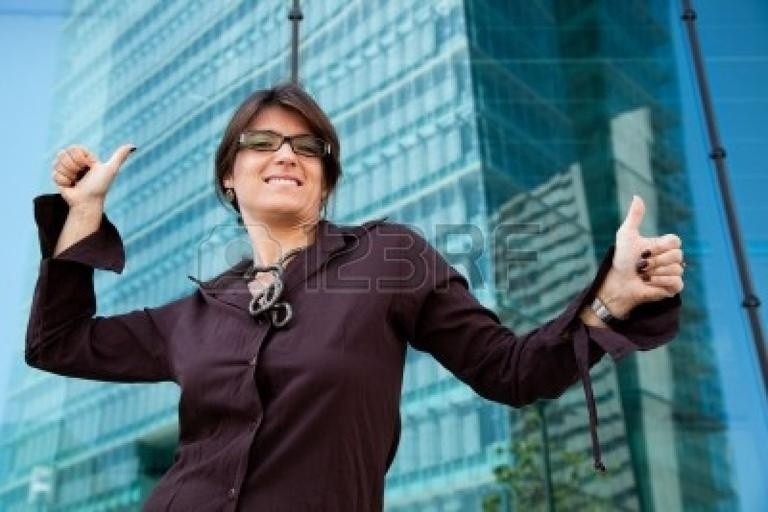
[236,129,331,157]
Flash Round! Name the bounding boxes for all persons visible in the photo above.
[23,81,688,512]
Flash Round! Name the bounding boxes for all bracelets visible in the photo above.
[590,296,629,338]
